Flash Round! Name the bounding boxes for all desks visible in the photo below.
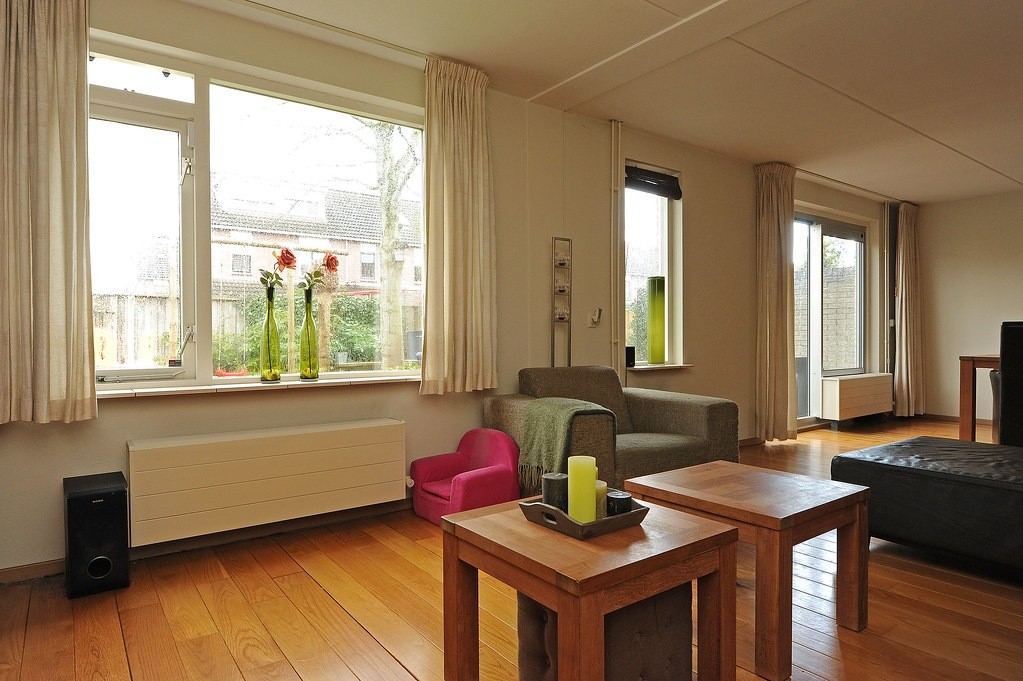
[623,459,870,681]
[440,494,741,681]
[958,354,1000,441]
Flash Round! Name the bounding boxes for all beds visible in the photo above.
[829,436,1023,568]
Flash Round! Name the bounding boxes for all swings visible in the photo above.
[214,283,248,377]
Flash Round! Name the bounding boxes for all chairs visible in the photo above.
[1000,321,1023,447]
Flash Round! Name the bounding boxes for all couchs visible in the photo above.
[482,366,740,499]
[409,428,520,525]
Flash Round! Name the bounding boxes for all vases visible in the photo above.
[261,288,280,382]
[299,289,320,380]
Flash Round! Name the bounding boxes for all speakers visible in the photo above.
[62,471,130,599]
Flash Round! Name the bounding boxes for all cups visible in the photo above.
[607,491,632,516]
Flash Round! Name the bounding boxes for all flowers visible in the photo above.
[298,250,339,373]
[257,247,298,373]
